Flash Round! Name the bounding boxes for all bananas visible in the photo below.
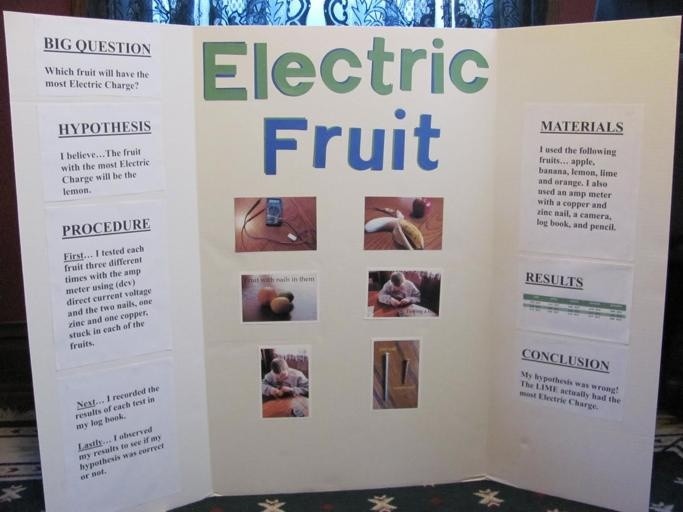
[365,208,424,250]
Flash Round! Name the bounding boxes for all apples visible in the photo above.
[413,198,431,218]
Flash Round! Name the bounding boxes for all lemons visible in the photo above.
[279,292,293,302]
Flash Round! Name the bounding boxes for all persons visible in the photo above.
[376,271,421,307]
[262,357,308,397]
